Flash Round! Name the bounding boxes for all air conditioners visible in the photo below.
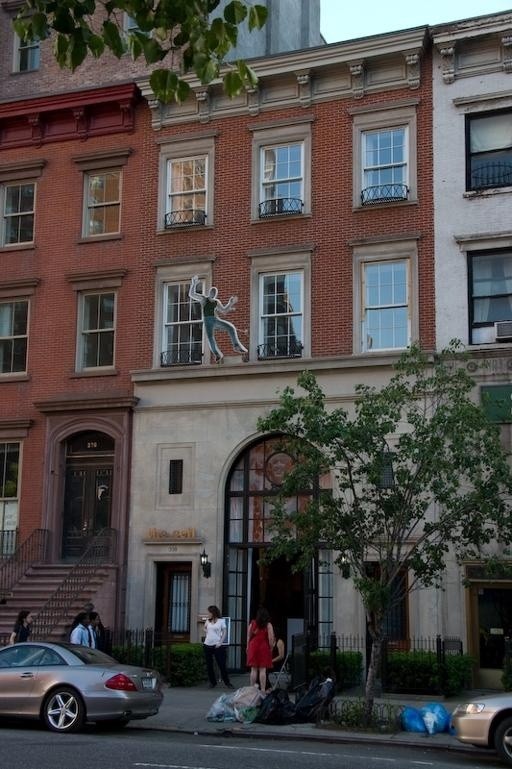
[495,321,512,339]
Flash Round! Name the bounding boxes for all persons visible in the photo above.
[69,611,99,649]
[189,275,248,362]
[203,605,233,689]
[246,607,284,693]
[9,610,32,644]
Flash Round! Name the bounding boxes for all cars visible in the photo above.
[0,641,163,733]
[450,692,512,764]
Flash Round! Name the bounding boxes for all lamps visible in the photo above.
[200,546,211,579]
[338,552,350,579]
